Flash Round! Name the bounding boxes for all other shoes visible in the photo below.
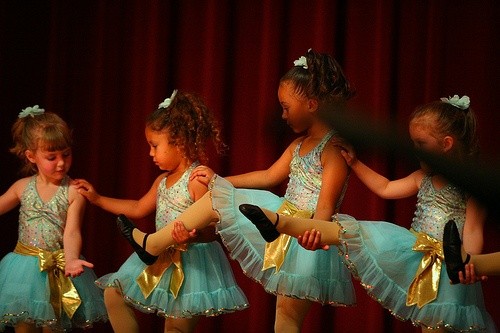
[117,214,160,265]
[238,204,280,242]
[443,219,470,284]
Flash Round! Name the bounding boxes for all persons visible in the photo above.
[0,105,110,333]
[239,93,498,332]
[442,219,500,284]
[117,47,360,333]
[72,87,250,333]
